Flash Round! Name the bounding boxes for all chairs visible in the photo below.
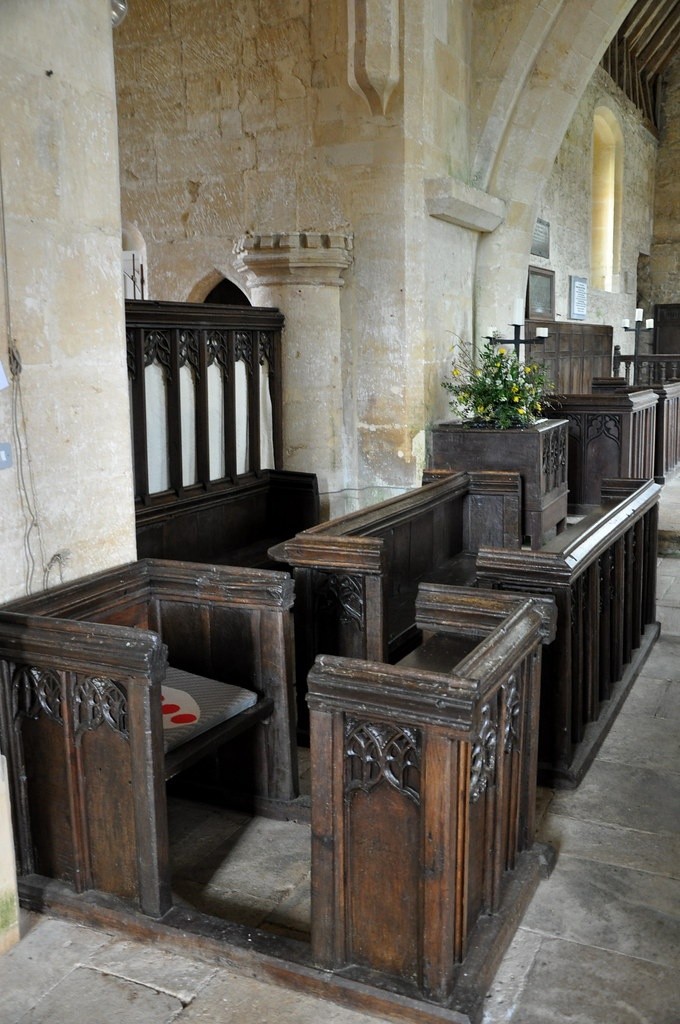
[0,558,298,917]
[304,582,558,996]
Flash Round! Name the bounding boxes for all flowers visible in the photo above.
[440,326,567,428]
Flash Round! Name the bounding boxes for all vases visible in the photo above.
[432,417,570,551]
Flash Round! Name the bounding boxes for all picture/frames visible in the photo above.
[528,265,555,321]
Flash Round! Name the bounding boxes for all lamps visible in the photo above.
[635,308,643,321]
[482,326,492,337]
[536,327,548,337]
[622,319,629,327]
[646,319,654,329]
[513,300,524,323]
